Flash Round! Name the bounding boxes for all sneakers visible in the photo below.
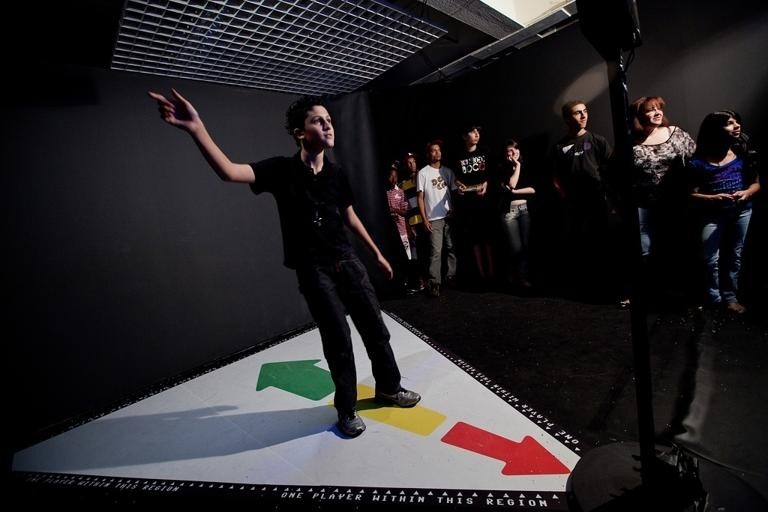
[728,302,746,314]
[405,276,440,298]
[338,408,366,436]
[375,383,421,407]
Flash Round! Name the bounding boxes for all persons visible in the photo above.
[386,125,537,297]
[148,86,422,437]
[552,99,631,309]
[628,95,698,303]
[683,108,761,314]
